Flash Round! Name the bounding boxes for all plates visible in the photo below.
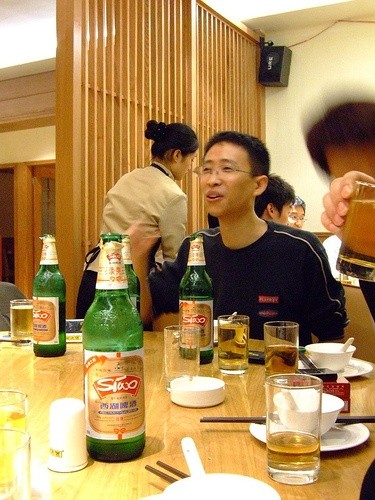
[250,421,370,452]
[297,358,374,378]
[160,473,281,500]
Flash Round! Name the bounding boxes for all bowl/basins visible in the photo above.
[214,319,231,344]
[305,343,357,372]
[272,391,345,438]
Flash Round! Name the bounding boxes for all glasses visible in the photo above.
[288,216,309,224]
[193,167,253,178]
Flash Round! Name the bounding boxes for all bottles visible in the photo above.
[82,232,145,463]
[178,233,215,365]
[119,234,140,316]
[33,234,66,358]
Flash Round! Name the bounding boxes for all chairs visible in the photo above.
[0,282,28,332]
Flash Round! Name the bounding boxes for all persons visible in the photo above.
[209,97,375,500]
[76,121,199,332]
[128,131,350,347]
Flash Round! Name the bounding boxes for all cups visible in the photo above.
[218,315,250,375]
[10,299,33,345]
[163,325,200,391]
[265,374,323,486]
[46,397,88,473]
[0,389,33,500]
[263,322,299,386]
[336,180,375,282]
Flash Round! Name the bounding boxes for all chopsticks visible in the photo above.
[199,416,375,424]
[145,460,189,484]
[299,352,321,374]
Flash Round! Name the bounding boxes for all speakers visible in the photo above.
[258,46,293,87]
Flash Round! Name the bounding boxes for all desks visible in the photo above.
[0,328,375,500]
